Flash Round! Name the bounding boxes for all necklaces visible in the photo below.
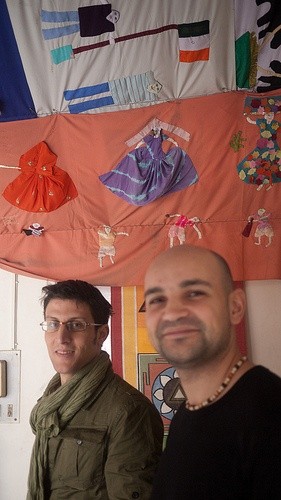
[184,355,247,411]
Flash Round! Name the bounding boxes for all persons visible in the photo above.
[24,278,164,500]
[147,245,281,500]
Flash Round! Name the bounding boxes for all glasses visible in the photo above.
[40,319,103,332]
